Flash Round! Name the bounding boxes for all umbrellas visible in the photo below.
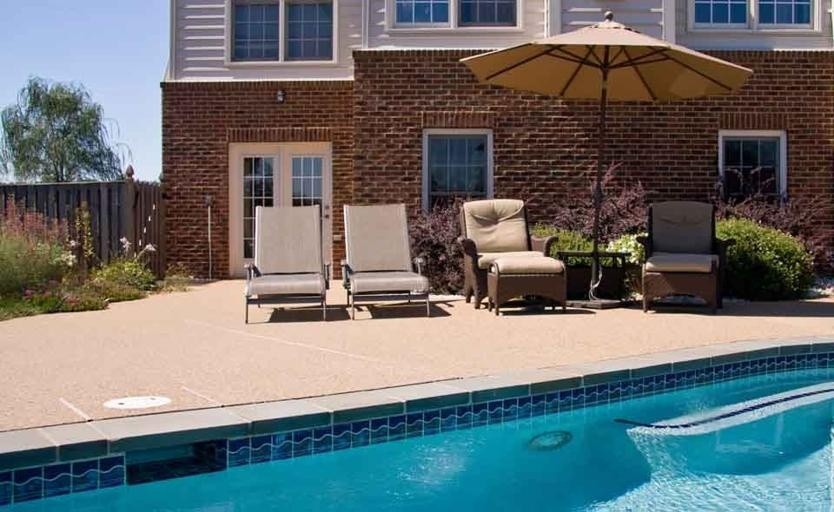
[457,11,753,290]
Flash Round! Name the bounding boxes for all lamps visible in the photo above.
[273,86,285,104]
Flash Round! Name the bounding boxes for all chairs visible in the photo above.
[636,198,725,310]
[341,196,432,320]
[244,202,332,326]
[459,196,566,316]
